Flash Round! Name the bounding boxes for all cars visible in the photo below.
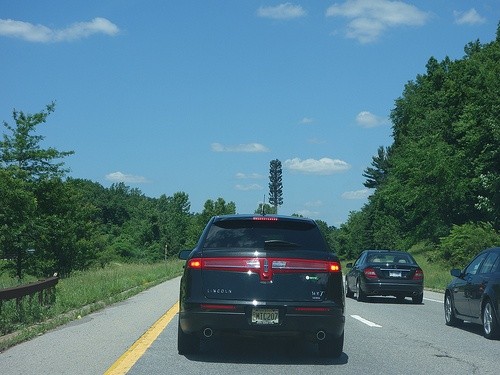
[444,247,500,340]
[345,250,424,303]
[178,214,345,359]
